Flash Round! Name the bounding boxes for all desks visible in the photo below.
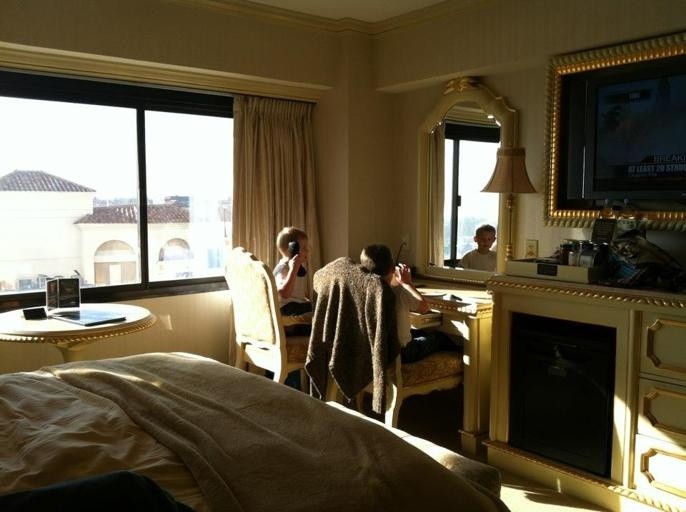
[411,279,493,462]
[1,303,158,363]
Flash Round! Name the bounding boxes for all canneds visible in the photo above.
[560,239,610,267]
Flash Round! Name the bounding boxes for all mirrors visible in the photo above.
[415,78,518,285]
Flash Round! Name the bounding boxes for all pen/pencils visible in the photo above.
[452,294,462,301]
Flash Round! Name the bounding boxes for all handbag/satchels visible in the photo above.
[596,230,686,291]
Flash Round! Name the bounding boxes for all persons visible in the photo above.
[360,242,463,366]
[272,226,315,338]
[457,223,496,273]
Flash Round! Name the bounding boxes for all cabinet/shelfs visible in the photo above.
[481,278,686,512]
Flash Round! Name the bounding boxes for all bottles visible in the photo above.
[600,199,635,218]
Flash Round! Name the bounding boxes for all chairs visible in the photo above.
[221,248,310,394]
[311,257,463,427]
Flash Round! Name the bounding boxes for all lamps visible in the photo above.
[481,147,539,275]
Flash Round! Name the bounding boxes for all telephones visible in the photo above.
[289,243,308,277]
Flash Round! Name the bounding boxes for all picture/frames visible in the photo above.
[543,30,686,231]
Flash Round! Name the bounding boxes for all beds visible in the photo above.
[1,350,510,512]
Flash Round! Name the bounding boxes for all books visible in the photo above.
[22,308,48,321]
[43,273,80,315]
[48,307,127,327]
